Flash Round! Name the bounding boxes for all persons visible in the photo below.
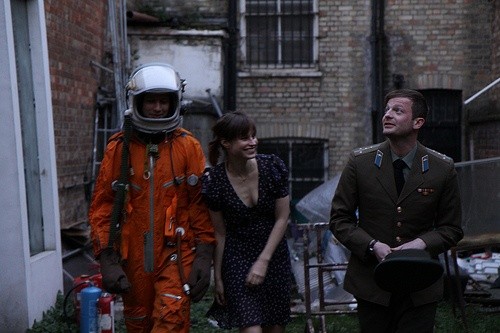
[329,89,464,333]
[201,112,292,333]
[89,62,217,333]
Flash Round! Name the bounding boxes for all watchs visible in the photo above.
[369,241,377,255]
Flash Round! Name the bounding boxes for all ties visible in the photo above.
[392,158,408,198]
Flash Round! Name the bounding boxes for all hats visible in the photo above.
[374,249,445,294]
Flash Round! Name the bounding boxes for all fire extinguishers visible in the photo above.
[75,274,92,323]
[97,295,114,333]
[80,281,102,333]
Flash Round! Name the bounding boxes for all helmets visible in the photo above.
[122,63,188,137]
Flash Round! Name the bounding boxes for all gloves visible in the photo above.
[187,241,216,303]
[95,247,133,295]
